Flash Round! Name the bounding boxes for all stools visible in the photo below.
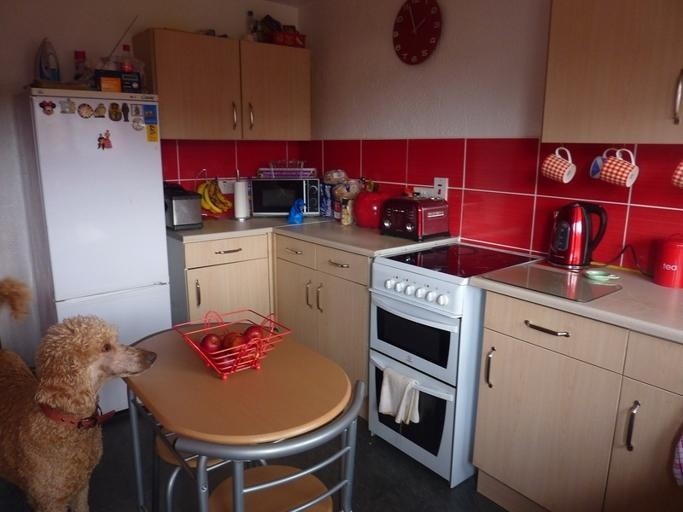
[146,430,266,512]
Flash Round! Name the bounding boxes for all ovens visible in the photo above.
[365,288,482,489]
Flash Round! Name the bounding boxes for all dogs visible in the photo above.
[0,277,158,512]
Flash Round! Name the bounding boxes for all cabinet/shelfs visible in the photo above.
[130,27,313,141]
[474,293,626,511]
[539,0,682,146]
[273,226,369,426]
[598,328,682,511]
[166,218,273,328]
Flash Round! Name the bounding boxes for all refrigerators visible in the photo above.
[0,88,174,417]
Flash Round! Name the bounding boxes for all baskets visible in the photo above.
[171,307,290,380]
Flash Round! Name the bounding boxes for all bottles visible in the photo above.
[354,190,382,229]
[71,49,98,91]
[120,44,133,72]
[245,10,257,41]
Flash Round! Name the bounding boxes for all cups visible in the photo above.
[599,148,639,188]
[671,160,683,190]
[272,32,300,47]
[590,147,621,179]
[541,147,577,185]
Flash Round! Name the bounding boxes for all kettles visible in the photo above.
[547,201,608,269]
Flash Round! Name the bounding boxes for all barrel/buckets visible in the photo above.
[37,37,60,80]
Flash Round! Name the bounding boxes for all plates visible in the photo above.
[583,270,619,282]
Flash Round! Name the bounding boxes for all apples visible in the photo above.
[199,325,263,354]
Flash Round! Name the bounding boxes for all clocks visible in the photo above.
[389,1,442,64]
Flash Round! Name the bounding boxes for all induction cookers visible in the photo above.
[374,238,543,309]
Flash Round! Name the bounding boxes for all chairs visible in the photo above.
[173,380,365,512]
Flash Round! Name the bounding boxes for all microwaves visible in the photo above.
[248,175,320,218]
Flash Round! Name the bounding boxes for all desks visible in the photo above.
[122,321,351,510]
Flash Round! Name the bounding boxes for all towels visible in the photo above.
[377,367,419,425]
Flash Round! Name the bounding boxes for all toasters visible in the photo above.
[162,184,203,232]
[380,197,449,240]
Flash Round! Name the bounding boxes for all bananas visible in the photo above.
[197,180,233,214]
